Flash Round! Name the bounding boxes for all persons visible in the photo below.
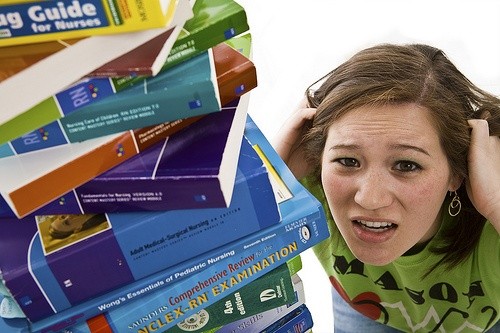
[275,44,499,333]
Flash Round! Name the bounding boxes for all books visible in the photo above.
[0,0,330,333]
